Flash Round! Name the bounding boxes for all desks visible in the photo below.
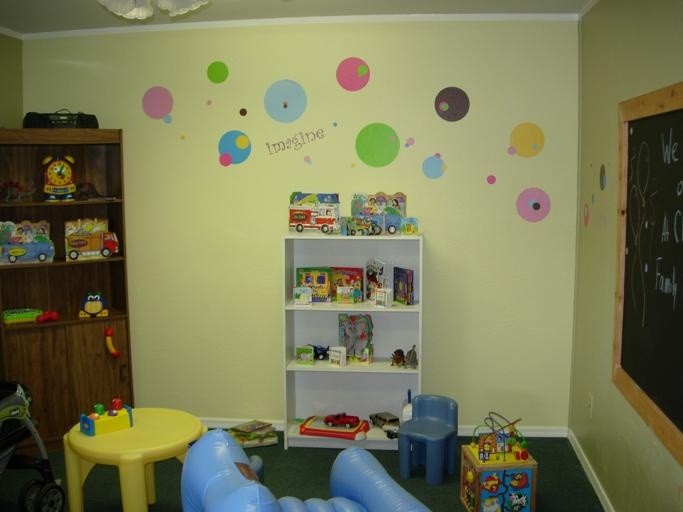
[62,406,209,512]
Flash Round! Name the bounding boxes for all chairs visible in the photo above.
[397,393,458,485]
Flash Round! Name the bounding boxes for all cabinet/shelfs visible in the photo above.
[282,232,424,450]
[0,126,136,451]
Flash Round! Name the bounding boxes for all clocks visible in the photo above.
[41,153,76,186]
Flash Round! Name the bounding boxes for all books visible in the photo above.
[292,258,415,308]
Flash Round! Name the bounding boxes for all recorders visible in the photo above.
[24,109,100,130]
